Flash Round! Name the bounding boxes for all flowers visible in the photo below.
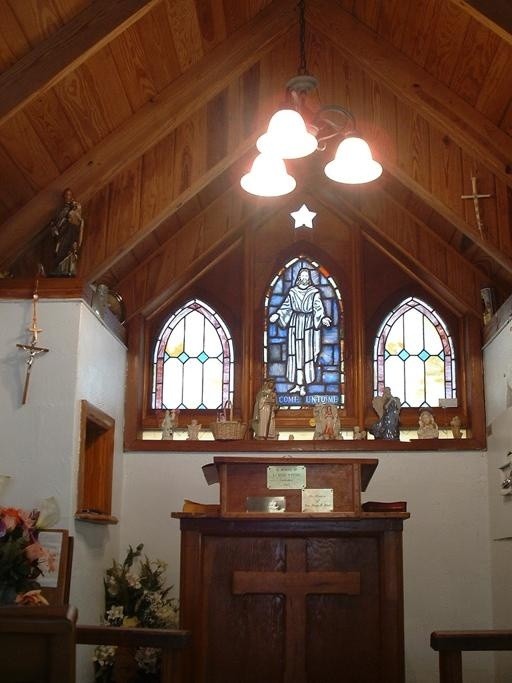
[1,503,58,606]
[90,542,182,677]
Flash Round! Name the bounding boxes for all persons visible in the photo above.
[161,409,175,440]
[46,187,84,276]
[269,267,332,397]
[323,405,335,436]
[250,378,279,441]
[417,411,439,439]
[369,387,401,440]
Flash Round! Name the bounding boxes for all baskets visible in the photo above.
[208,400,249,440]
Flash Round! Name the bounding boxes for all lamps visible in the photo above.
[237,2,384,197]
